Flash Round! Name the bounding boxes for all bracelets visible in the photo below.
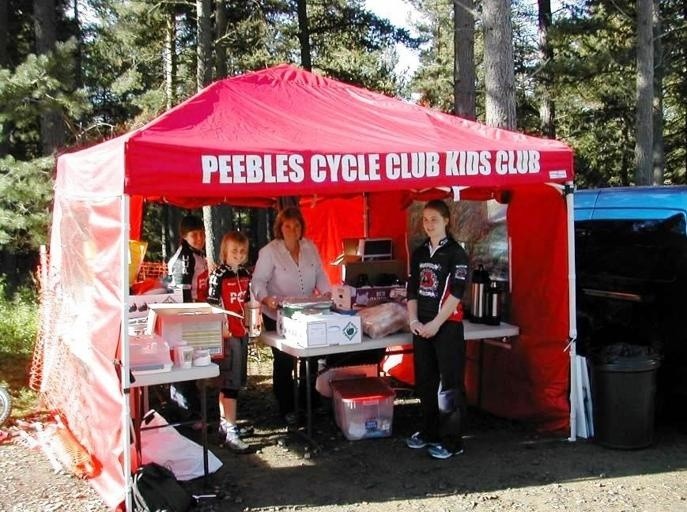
[409,319,421,325]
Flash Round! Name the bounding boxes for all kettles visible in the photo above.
[467,265,502,322]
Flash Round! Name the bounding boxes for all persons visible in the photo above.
[164,215,211,431]
[205,232,252,454]
[252,202,334,426]
[404,199,468,459]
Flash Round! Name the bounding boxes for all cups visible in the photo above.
[178,347,193,369]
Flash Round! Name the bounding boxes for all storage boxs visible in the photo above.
[328,376,393,441]
[148,300,222,357]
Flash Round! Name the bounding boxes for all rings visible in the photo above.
[272,300,276,305]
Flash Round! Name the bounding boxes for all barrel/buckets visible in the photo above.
[586,341,667,451]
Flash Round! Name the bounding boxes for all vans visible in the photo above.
[461,189,687,367]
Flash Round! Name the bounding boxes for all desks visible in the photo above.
[129,362,220,491]
[254,317,521,455]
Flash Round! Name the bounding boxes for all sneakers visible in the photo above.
[406,431,440,449]
[429,445,463,459]
[225,434,249,453]
[219,423,252,437]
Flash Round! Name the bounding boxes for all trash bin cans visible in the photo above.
[598,358,660,450]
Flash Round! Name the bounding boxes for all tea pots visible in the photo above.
[244,301,263,338]
